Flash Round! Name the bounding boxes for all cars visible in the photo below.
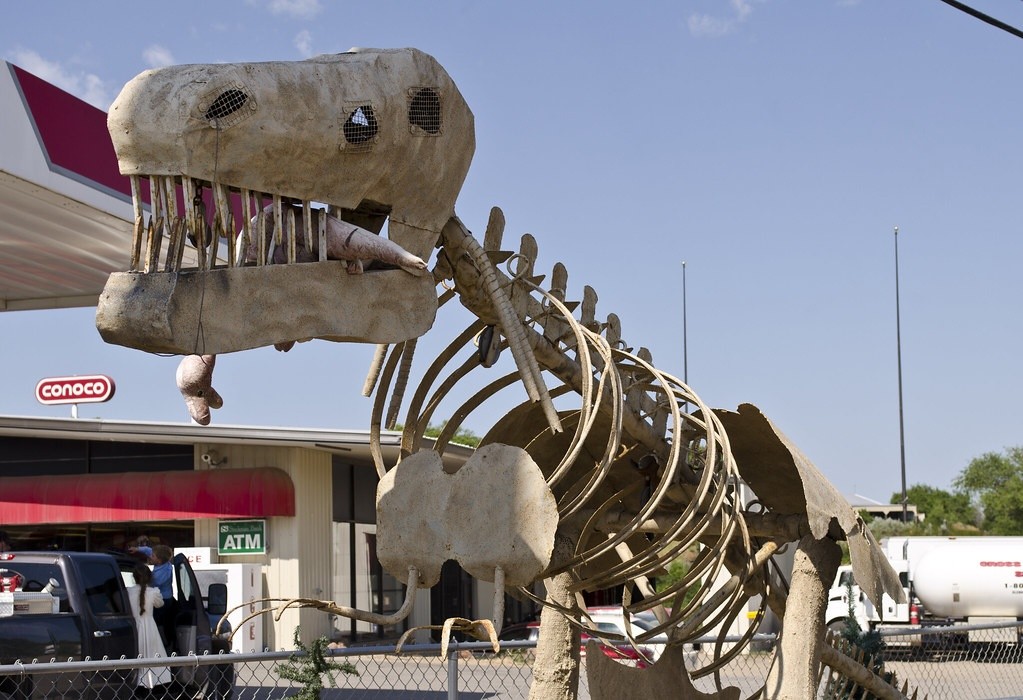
[487,605,699,670]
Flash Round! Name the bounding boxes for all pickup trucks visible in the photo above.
[1,549,239,700]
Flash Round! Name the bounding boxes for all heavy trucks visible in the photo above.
[823,534,1023,665]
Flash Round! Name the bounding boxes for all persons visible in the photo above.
[125,565,171,689]
[128,544,182,680]
